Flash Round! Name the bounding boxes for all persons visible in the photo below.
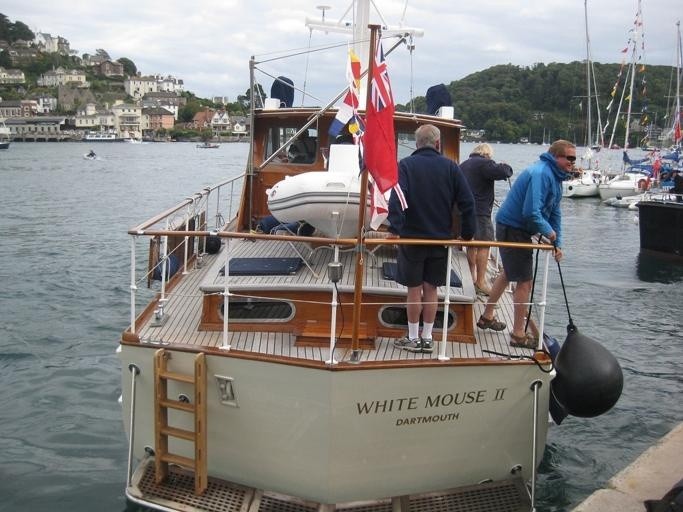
[297,129,318,157]
[458,143,511,295]
[476,140,584,348]
[87,148,96,157]
[388,123,477,352]
[287,143,317,163]
[593,160,600,178]
[653,157,662,180]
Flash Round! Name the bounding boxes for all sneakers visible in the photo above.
[392,334,423,353]
[420,334,434,353]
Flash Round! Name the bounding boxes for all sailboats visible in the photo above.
[599,15,655,199]
[112,0,559,511]
[561,15,604,197]
[0,94,12,149]
[656,20,683,191]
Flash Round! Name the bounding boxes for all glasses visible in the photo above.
[559,155,577,162]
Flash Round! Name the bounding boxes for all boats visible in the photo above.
[196,140,221,149]
[603,191,683,210]
[83,149,100,162]
[264,171,375,252]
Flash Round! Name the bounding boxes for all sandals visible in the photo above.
[509,332,540,349]
[474,280,491,296]
[476,314,507,331]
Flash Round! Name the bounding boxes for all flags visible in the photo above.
[364,30,398,194]
[327,47,362,138]
[353,109,408,232]
